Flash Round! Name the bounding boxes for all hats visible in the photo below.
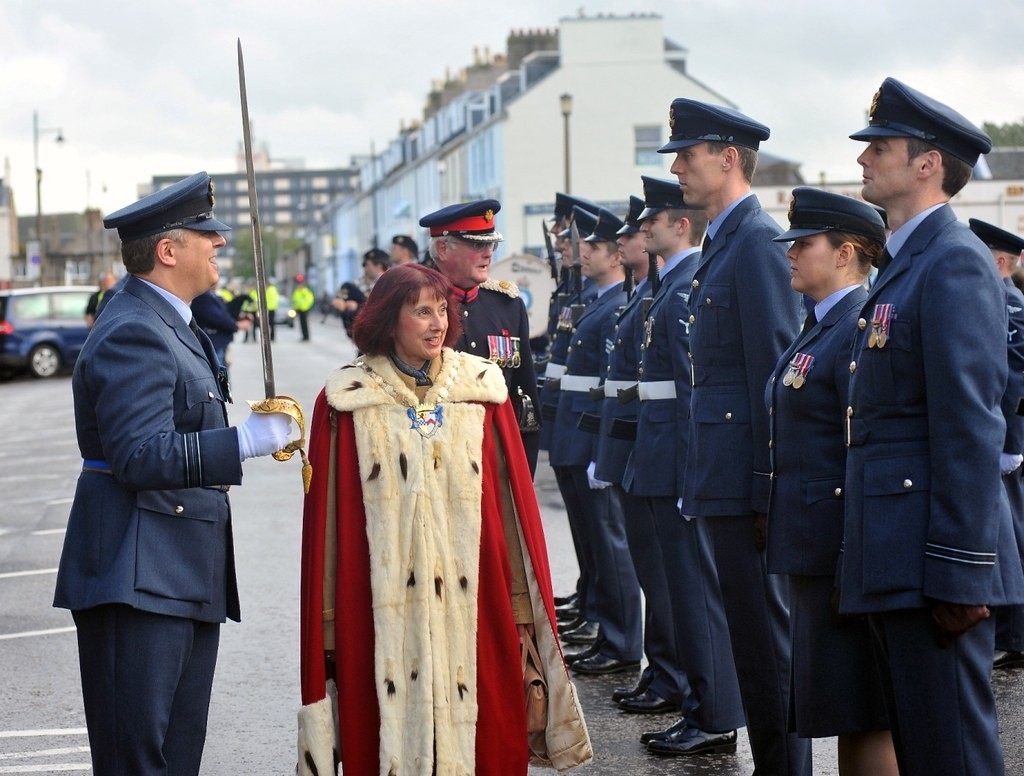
[419,199,504,243]
[615,195,646,234]
[656,97,770,152]
[848,76,992,166]
[584,209,624,242]
[772,187,884,242]
[556,205,598,238]
[636,175,704,221]
[361,235,420,268]
[549,191,598,222]
[968,216,1024,256]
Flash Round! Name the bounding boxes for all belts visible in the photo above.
[81,459,231,492]
[542,362,678,401]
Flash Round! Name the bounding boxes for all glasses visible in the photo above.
[466,238,498,252]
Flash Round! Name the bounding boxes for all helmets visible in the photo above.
[101,170,232,245]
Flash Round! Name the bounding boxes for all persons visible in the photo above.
[416,200,538,488]
[297,262,536,776]
[49,171,293,776]
[82,236,421,358]
[536,77,1024,775]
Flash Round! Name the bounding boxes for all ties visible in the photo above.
[876,247,893,278]
[592,293,598,302]
[652,273,662,298]
[701,234,712,257]
[801,309,817,339]
[190,315,203,346]
[631,290,636,299]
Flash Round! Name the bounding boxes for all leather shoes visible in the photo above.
[991,651,1024,669]
[551,591,739,757]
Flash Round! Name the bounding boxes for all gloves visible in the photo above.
[587,462,613,489]
[676,498,698,522]
[236,410,292,462]
[999,453,1023,476]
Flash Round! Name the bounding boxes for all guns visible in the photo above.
[538,218,663,302]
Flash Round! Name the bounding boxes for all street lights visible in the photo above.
[34,111,63,284]
[561,95,573,195]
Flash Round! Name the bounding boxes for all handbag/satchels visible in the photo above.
[521,630,549,733]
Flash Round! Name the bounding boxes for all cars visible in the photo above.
[272,296,296,327]
[0,285,101,379]
